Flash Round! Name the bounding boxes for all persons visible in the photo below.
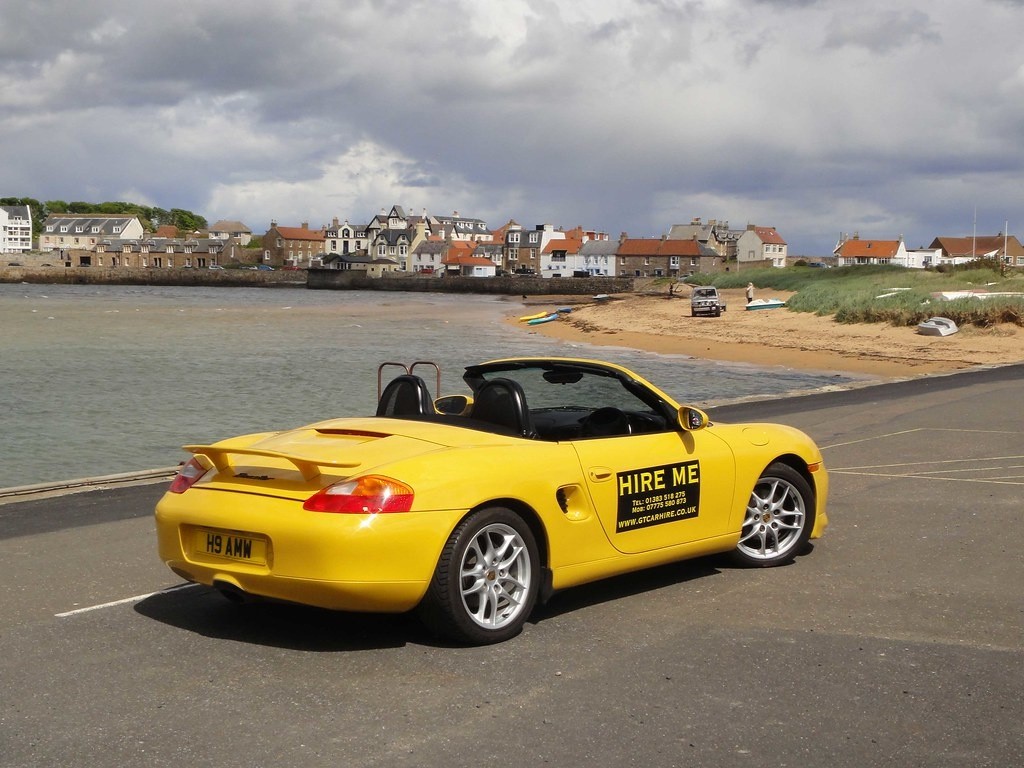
[669,282,673,295]
[746,282,755,303]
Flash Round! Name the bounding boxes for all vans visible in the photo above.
[689,287,722,317]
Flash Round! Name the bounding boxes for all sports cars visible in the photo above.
[155,357,830,647]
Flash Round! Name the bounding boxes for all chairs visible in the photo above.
[471,376,529,432]
[375,374,436,415]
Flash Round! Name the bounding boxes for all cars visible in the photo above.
[258,265,275,271]
[678,274,690,284]
[78,264,89,267]
[806,262,826,268]
[208,265,225,270]
[239,264,257,271]
[290,266,302,270]
[8,262,20,266]
[41,263,55,267]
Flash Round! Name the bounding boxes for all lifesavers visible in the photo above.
[520,307,571,324]
